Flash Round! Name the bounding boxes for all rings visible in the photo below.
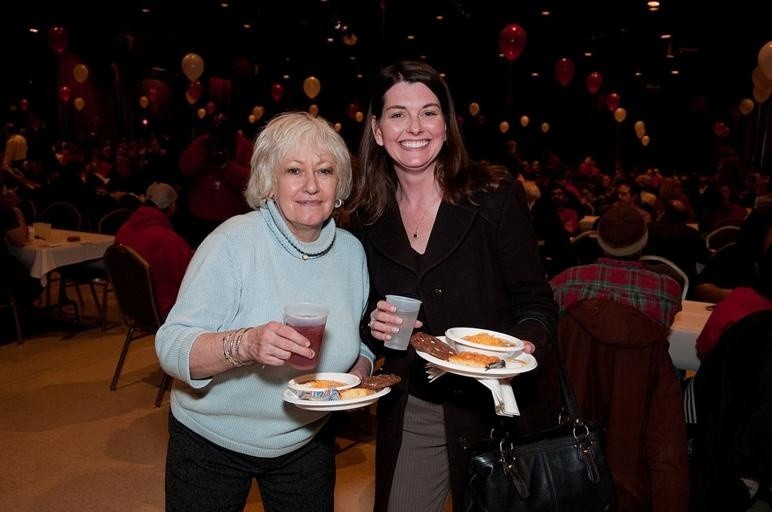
[368,319,375,328]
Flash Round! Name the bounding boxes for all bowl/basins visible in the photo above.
[444,326,526,361]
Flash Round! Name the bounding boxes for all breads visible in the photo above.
[339,385,377,402]
[450,350,500,368]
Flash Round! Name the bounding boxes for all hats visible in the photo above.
[596,206,649,257]
[145,182,178,210]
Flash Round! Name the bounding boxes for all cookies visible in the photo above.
[410,330,455,362]
[359,373,402,389]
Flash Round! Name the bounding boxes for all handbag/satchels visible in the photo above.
[461,414,616,510]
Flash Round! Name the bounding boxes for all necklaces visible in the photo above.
[390,191,442,244]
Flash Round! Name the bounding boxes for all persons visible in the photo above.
[1,123,772,362]
[152,108,376,512]
[339,60,562,511]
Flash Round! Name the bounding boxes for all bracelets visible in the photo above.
[222,326,255,369]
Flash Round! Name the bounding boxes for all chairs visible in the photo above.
[545,223,770,302]
[99,242,179,411]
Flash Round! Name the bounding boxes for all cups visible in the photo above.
[383,293,421,351]
[283,301,331,372]
[33,222,63,246]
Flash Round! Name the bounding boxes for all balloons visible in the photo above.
[20,21,772,155]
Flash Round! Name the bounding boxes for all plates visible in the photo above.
[415,336,538,380]
[287,372,361,393]
[283,385,391,412]
